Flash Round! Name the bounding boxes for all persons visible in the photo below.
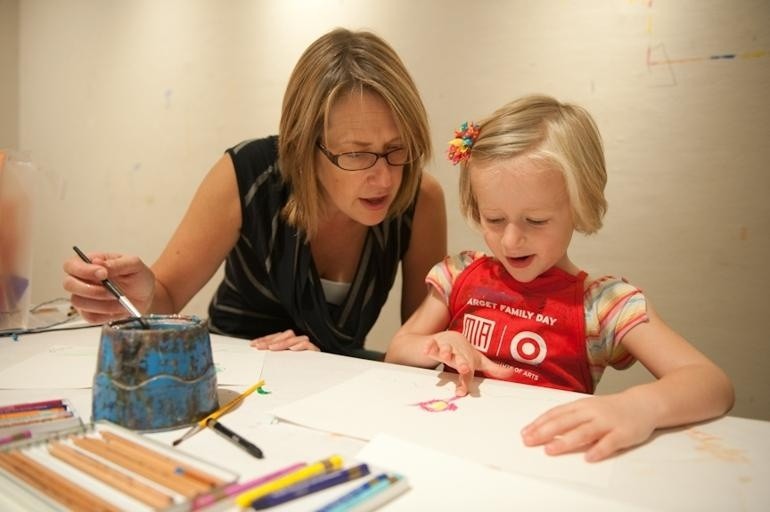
[383,97,736,463]
[62,26,448,362]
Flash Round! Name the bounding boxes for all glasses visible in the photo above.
[316,137,422,170]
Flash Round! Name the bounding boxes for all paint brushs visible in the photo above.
[73,246,151,329]
[172,379,265,448]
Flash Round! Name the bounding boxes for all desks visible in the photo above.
[3,316,770,512]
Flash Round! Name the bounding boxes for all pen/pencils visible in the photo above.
[191,456,414,511]
[207,418,264,459]
[2,400,235,510]
